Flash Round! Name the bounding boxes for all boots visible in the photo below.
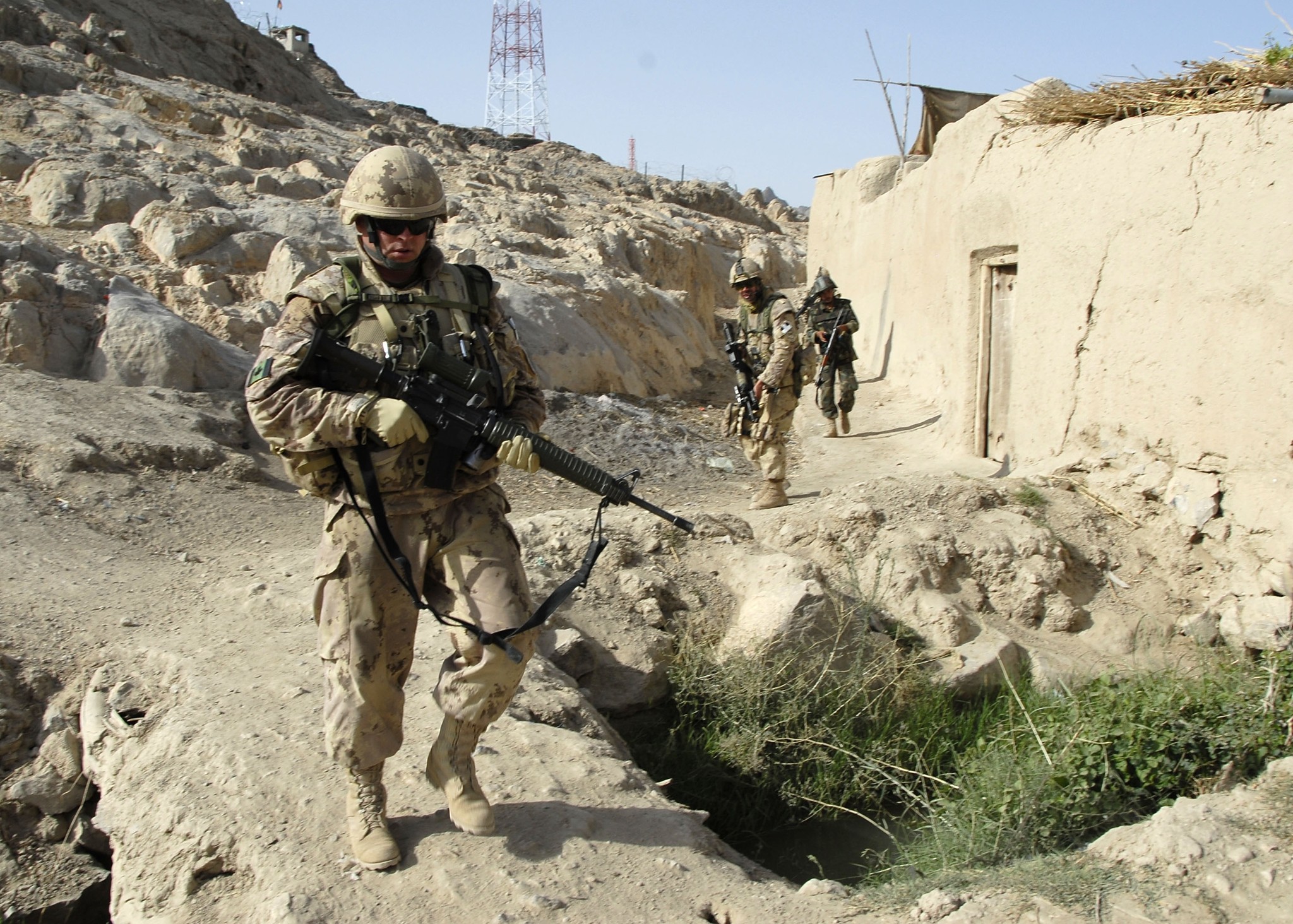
[747,478,791,510]
[840,410,851,434]
[426,711,497,837]
[345,759,402,870]
[823,418,837,438]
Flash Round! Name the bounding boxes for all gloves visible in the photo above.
[360,398,430,447]
[496,435,540,473]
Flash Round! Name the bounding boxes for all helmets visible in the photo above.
[729,257,762,288]
[809,275,838,296]
[338,145,448,225]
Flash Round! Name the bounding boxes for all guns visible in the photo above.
[722,320,777,424]
[814,302,851,387]
[298,325,694,664]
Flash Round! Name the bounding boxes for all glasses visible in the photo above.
[365,215,436,236]
[734,278,761,291]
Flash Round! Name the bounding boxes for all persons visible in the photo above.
[242,145,547,872]
[720,257,800,511]
[799,276,860,438]
